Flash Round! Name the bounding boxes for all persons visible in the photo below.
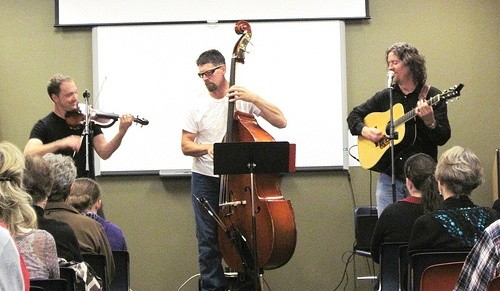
[1,73,135,290]
[181,50,288,291]
[347,42,500,291]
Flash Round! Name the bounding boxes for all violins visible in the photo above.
[65,102,150,128]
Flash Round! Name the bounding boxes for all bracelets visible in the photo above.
[425,122,436,127]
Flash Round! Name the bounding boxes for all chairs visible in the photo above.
[420,261,464,291]
[29,250,130,291]
[379,242,413,291]
[409,250,469,291]
[397,245,444,291]
[352,206,381,291]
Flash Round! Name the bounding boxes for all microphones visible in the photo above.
[387,71,395,89]
[83,90,91,98]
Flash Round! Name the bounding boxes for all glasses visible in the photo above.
[198,65,221,78]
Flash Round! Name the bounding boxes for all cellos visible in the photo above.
[217,20,297,273]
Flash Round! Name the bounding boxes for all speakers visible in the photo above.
[355,206,378,250]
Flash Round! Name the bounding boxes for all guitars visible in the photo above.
[357,83,465,173]
